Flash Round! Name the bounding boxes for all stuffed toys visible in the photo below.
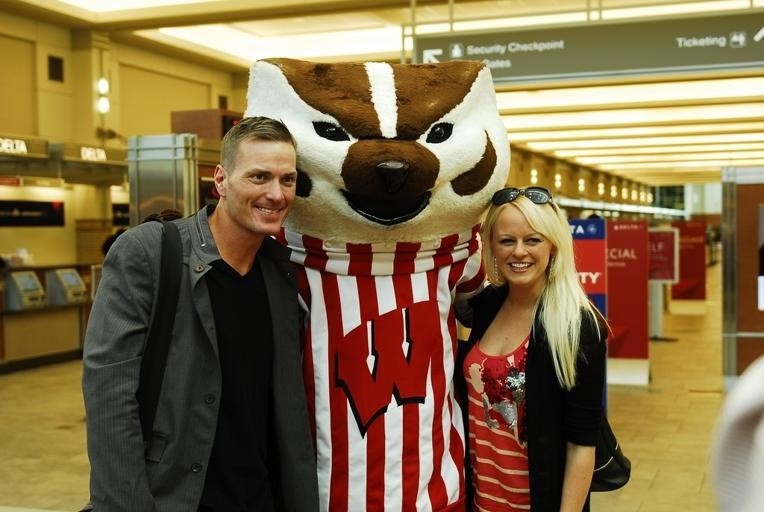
[238,56,514,512]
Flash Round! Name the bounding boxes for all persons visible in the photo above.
[80,113,321,512]
[451,185,612,512]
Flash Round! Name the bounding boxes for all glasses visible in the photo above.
[492,187,557,215]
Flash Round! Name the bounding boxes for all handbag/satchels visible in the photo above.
[589,415,630,492]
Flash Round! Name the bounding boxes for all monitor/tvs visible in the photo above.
[59,271,82,290]
[15,274,41,292]
